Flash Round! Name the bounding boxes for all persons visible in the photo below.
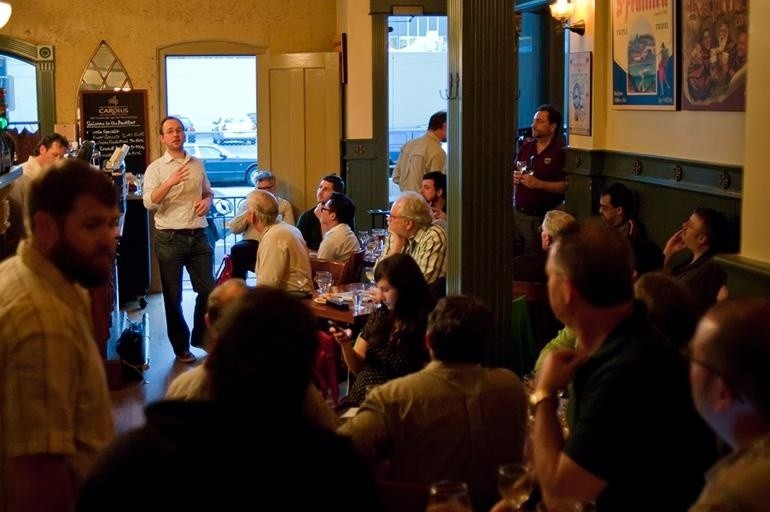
[419,169,448,220]
[661,206,726,295]
[512,104,569,250]
[385,191,448,286]
[532,217,706,512]
[1,158,124,512]
[331,294,529,498]
[316,192,361,267]
[532,273,690,397]
[296,174,356,252]
[687,295,770,512]
[228,170,295,280]
[656,41,671,96]
[244,189,315,295]
[142,116,216,363]
[392,110,448,194]
[537,209,577,252]
[686,22,748,97]
[597,180,665,271]
[163,277,342,432]
[329,252,437,416]
[7,132,70,251]
[78,283,388,512]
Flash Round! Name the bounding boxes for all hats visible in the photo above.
[542,210,575,237]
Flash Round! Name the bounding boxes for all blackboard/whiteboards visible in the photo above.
[75,89,150,174]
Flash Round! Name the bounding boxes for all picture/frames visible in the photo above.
[567,51,592,136]
[678,1,747,112]
[79,90,150,191]
[608,1,677,112]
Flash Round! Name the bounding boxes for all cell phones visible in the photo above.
[328,319,340,332]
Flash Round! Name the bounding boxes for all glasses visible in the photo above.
[537,225,544,232]
[321,207,330,212]
[256,185,274,191]
[678,345,745,406]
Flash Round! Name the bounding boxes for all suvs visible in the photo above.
[387,126,433,178]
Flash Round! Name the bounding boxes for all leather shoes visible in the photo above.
[175,350,196,363]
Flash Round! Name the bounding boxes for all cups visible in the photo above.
[367,242,377,258]
[358,231,367,244]
[315,271,331,296]
[349,283,364,309]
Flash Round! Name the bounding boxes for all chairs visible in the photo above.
[507,283,551,344]
[310,256,345,287]
[348,248,367,285]
[428,275,446,311]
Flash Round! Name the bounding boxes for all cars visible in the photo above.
[178,116,195,142]
[183,143,257,188]
[211,114,256,145]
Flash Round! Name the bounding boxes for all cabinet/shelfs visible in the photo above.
[115,190,151,309]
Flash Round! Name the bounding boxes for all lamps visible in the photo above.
[550,1,586,37]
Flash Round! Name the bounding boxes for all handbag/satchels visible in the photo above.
[115,319,143,382]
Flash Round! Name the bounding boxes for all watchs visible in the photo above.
[528,388,559,417]
